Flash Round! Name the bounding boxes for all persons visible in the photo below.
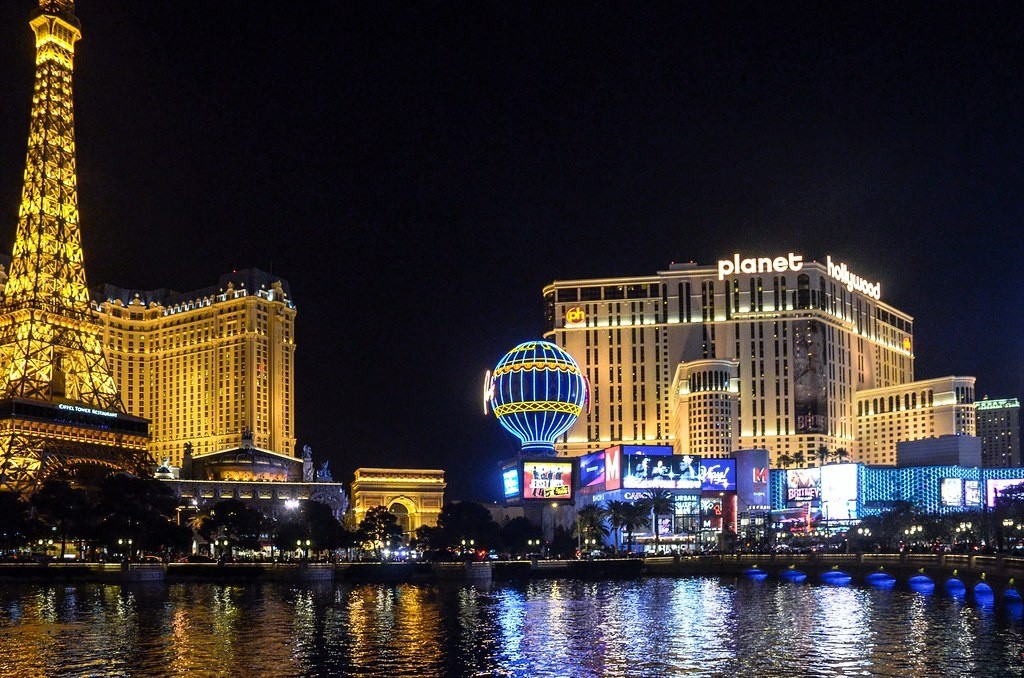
[532,466,562,497]
[680,456,699,480]
[636,460,650,481]
[652,460,668,480]
